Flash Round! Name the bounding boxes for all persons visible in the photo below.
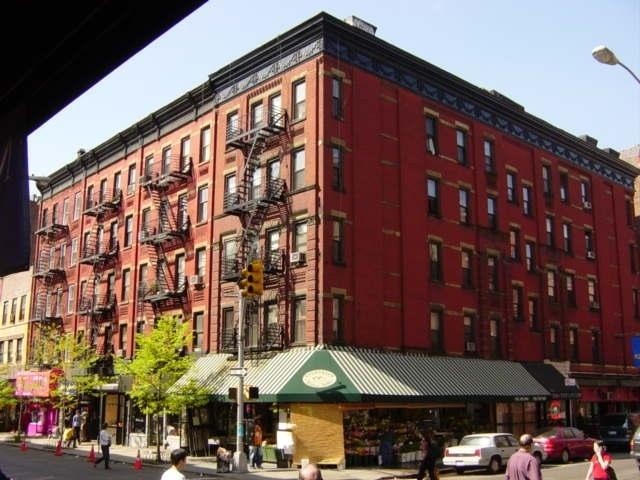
[418,431,431,480]
[585,439,618,480]
[504,433,543,480]
[160,448,193,480]
[298,463,324,480]
[94,422,117,469]
[72,410,85,445]
[251,419,264,469]
[427,429,441,480]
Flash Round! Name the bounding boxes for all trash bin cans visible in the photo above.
[217,454,230,473]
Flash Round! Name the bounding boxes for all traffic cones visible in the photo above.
[20,436,30,451]
[132,448,143,471]
[53,440,66,458]
[84,444,98,463]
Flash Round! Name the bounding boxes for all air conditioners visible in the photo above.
[588,251,596,258]
[190,274,203,285]
[289,252,305,263]
[584,201,592,209]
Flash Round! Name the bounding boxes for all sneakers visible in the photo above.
[94,462,112,469]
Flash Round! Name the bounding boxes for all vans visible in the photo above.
[596,412,640,447]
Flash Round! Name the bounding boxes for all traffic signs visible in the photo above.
[228,367,249,377]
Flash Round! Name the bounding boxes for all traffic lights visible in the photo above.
[246,257,266,296]
[242,385,259,401]
[239,268,247,298]
[228,388,236,399]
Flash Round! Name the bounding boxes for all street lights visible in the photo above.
[591,45,640,85]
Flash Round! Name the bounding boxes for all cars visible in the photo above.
[524,422,608,466]
[442,429,548,474]
[629,424,640,470]
